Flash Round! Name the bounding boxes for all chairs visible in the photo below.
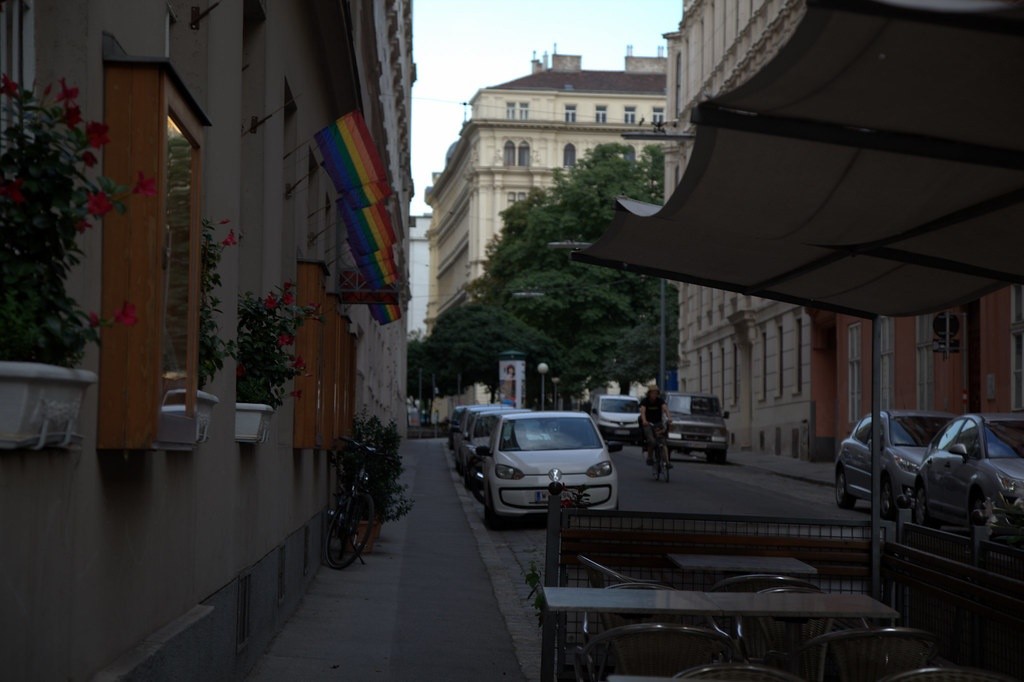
[572,554,1016,681]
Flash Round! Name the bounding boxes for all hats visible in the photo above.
[648,385,660,394]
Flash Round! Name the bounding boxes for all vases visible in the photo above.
[160,389,220,444]
[233,403,279,445]
[1,363,98,450]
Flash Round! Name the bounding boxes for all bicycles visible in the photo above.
[325,435,388,569]
[643,421,669,483]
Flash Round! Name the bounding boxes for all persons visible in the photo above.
[639,384,674,469]
[504,365,515,380]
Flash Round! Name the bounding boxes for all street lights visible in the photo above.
[537,363,549,411]
[552,378,559,409]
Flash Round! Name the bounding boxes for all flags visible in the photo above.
[314,107,403,327]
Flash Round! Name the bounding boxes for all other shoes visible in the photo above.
[666,463,673,468]
[646,457,653,465]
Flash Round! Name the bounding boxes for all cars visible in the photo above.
[448,405,531,489]
[913,413,1024,529]
[834,409,959,520]
[475,412,619,522]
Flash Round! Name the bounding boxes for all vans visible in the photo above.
[591,394,641,445]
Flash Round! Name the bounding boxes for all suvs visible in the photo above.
[659,392,730,462]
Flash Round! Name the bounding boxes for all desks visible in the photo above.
[666,553,817,582]
[540,586,902,676]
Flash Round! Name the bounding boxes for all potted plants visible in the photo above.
[327,404,416,552]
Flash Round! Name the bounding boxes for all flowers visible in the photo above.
[1,73,157,358]
[168,212,242,385]
[237,280,325,406]
